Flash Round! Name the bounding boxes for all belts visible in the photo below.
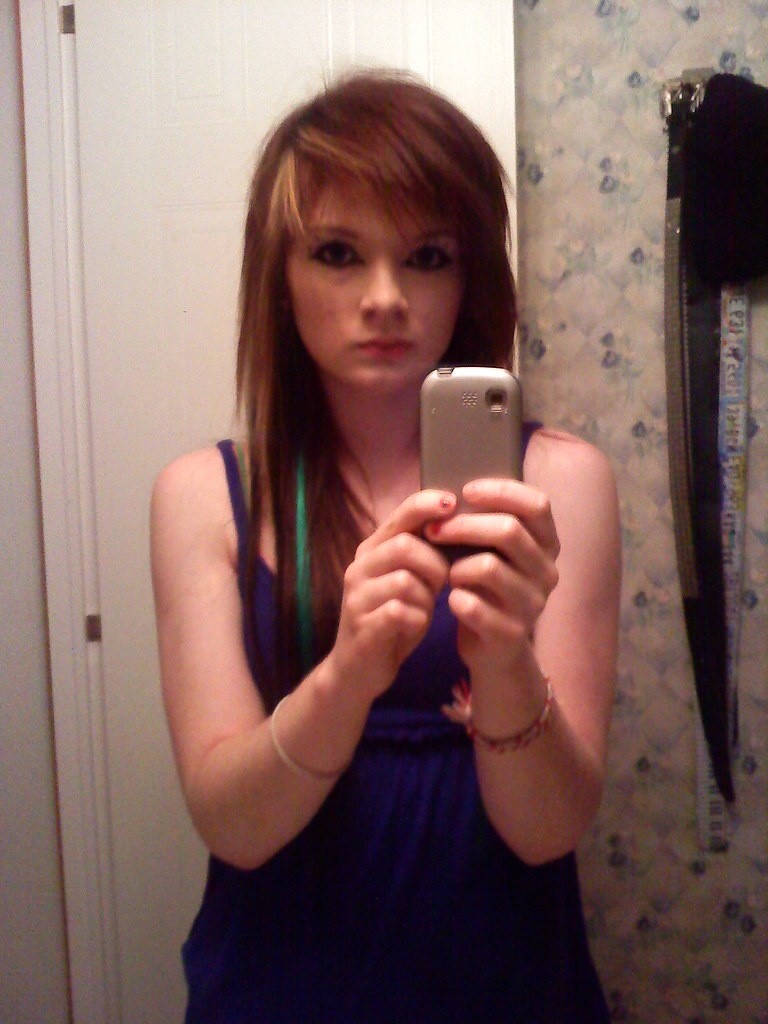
[660,79,740,807]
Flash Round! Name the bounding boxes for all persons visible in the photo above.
[149,71,623,1024]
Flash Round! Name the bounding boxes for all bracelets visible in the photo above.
[271,694,353,784]
[465,672,553,754]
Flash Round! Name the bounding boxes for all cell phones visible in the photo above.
[418,367,521,560]
